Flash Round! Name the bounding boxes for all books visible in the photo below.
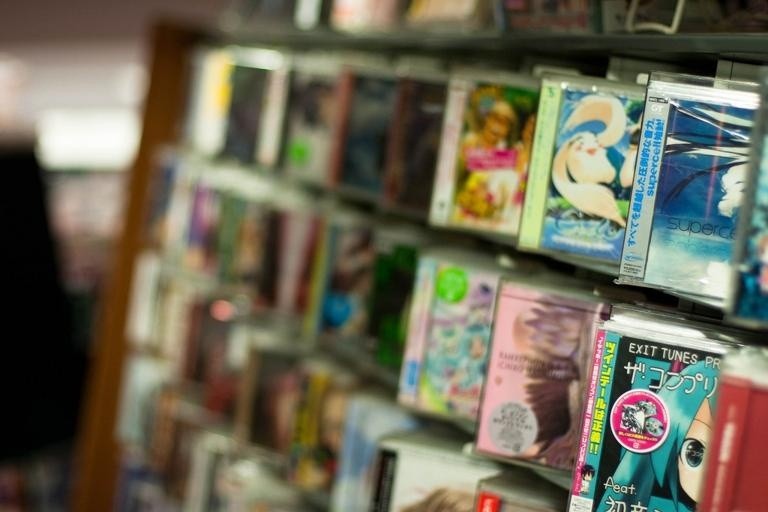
[398,252,503,419]
[428,65,541,236]
[476,275,612,465]
[514,78,645,267]
[619,78,761,294]
[114,344,394,511]
[128,45,428,342]
[381,440,498,510]
[564,316,739,512]
[699,358,768,512]
